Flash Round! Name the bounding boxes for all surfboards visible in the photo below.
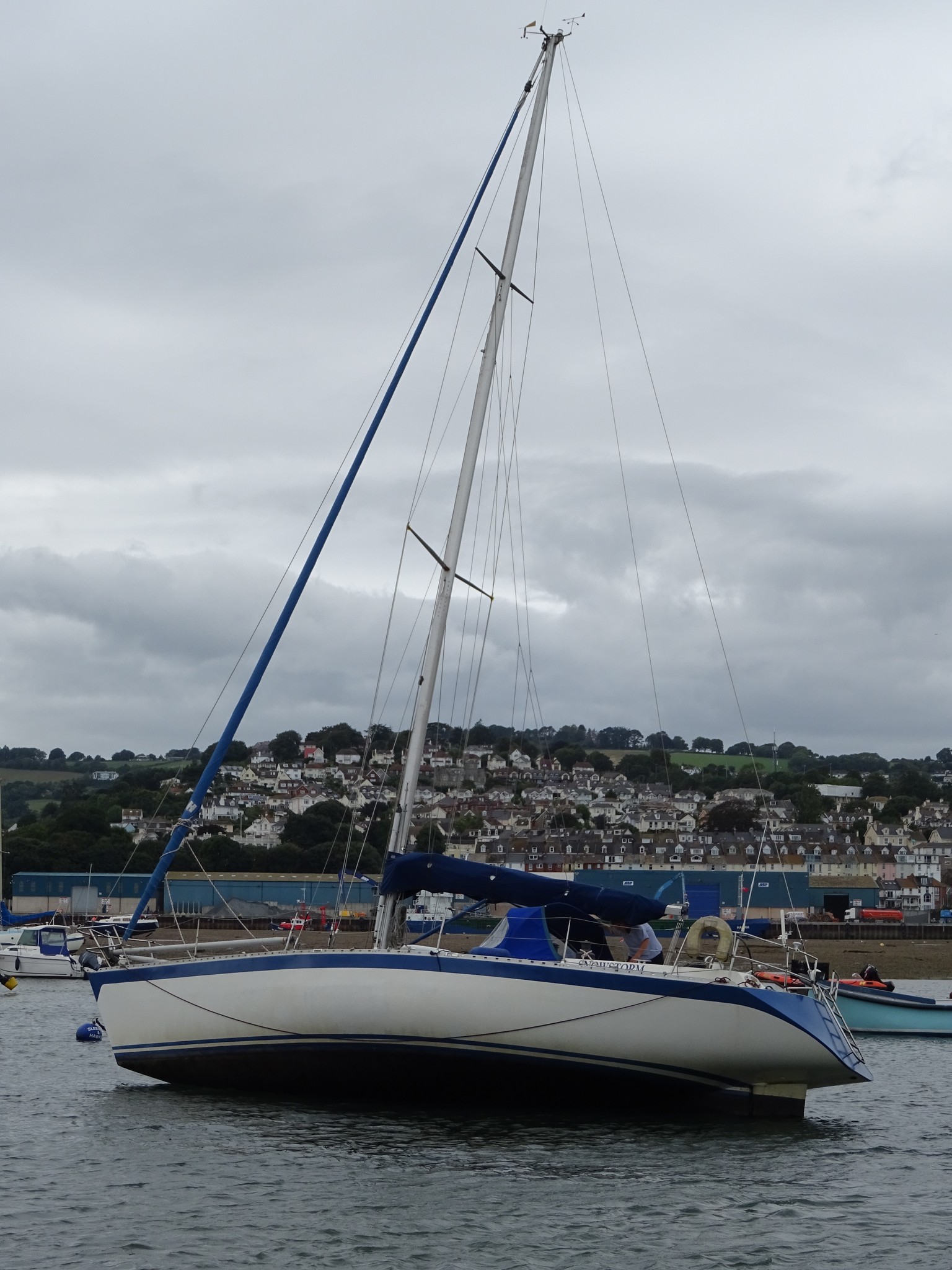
[754,971,887,989]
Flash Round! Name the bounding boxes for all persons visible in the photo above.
[602,915,664,965]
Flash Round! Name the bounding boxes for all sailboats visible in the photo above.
[66,2,878,1126]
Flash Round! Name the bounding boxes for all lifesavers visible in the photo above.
[686,916,733,961]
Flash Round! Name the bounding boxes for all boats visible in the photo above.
[749,961,951,1038]
[0,903,107,978]
[269,887,313,930]
[85,863,159,937]
